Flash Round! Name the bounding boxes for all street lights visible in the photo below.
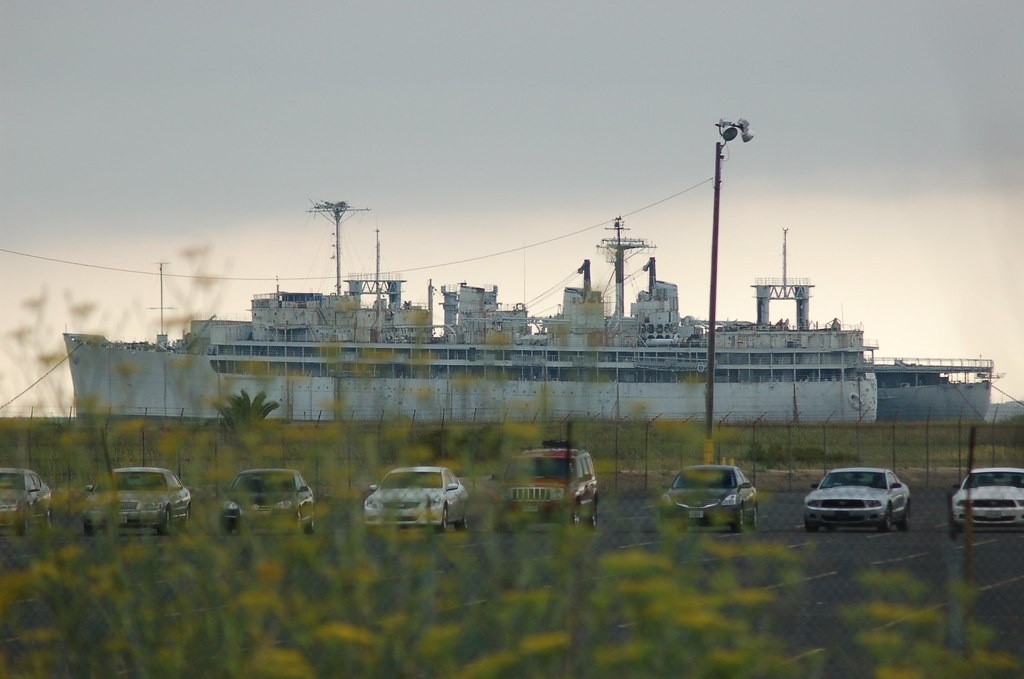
[705,117,754,463]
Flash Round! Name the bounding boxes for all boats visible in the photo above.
[870,356,994,421]
[62,200,878,426]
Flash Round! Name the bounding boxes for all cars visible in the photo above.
[951,468,1024,533]
[659,463,758,535]
[364,465,471,533]
[220,469,315,535]
[803,467,912,532]
[0,468,52,535]
[82,466,191,536]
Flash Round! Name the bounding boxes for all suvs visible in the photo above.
[480,439,598,530]
